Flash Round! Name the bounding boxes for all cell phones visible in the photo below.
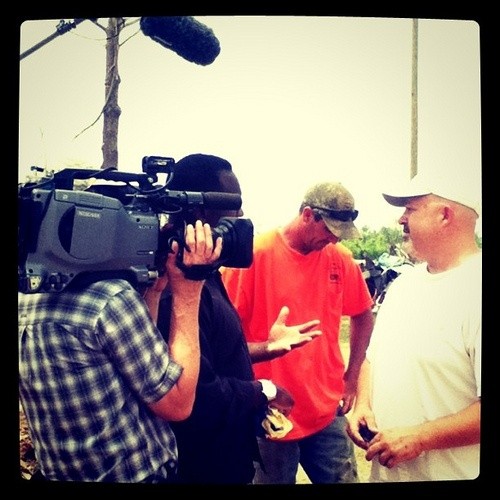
[359,426,375,442]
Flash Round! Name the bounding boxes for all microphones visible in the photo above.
[182,191,242,209]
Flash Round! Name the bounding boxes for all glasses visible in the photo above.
[310,206,359,222]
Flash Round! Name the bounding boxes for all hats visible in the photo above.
[303,181,362,239]
[382,168,479,219]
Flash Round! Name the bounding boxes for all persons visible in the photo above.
[135,154,321,483]
[20,221,223,484]
[219,180,375,484]
[345,164,482,482]
